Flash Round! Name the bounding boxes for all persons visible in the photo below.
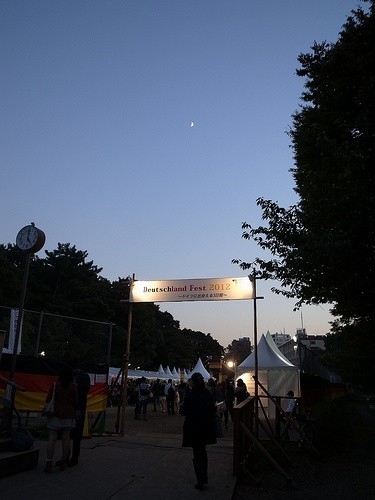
[108,375,176,422]
[177,376,247,432]
[280,391,295,426]
[179,373,217,493]
[55,358,90,467]
[43,366,79,473]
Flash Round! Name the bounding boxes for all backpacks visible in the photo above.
[11,422,34,453]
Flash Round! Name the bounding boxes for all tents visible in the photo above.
[266,331,295,367]
[234,334,299,441]
[187,357,217,387]
[158,364,189,385]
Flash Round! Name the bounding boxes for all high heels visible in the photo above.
[43,461,52,474]
[60,457,67,471]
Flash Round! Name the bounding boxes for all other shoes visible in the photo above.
[204,478,209,484]
[223,425,230,431]
[143,418,145,421]
[135,416,139,420]
[71,458,78,465]
[194,483,204,492]
[138,417,141,420]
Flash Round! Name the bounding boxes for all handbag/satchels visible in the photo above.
[215,400,227,414]
[214,416,224,438]
[41,381,56,418]
[168,383,176,397]
[140,389,150,396]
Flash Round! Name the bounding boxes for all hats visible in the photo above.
[287,391,294,396]
[156,379,160,382]
